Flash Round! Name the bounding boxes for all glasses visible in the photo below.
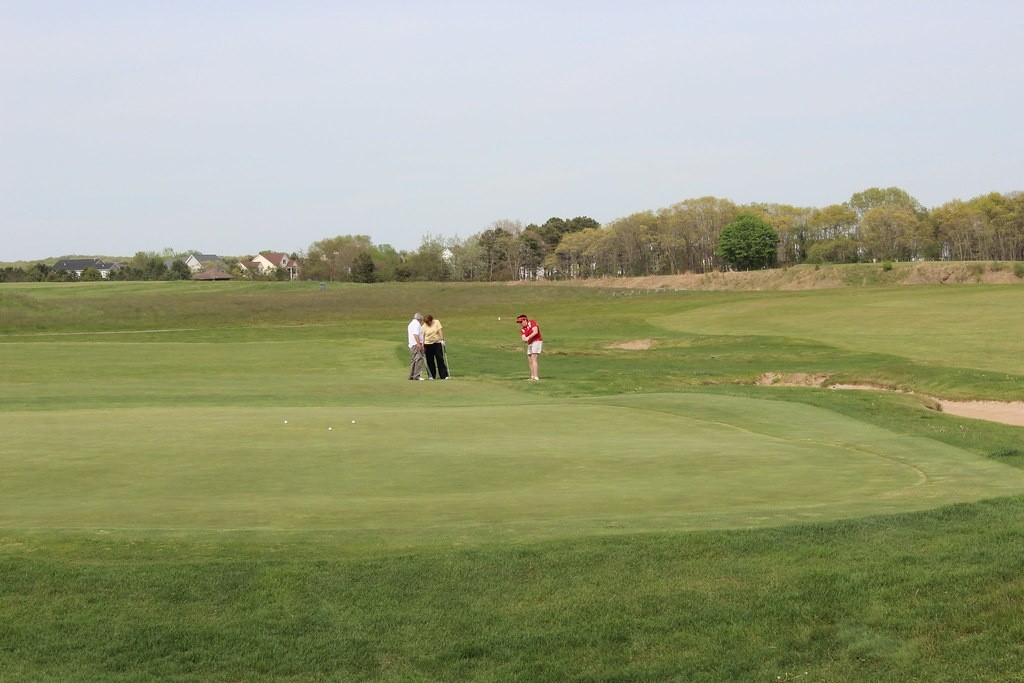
[520,321,523,323]
[425,320,429,322]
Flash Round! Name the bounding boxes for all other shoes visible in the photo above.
[429,377,435,380]
[416,377,425,380]
[445,376,450,380]
[527,376,539,381]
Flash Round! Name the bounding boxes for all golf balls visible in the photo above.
[351,420,356,424]
[284,421,287,424]
[498,317,501,321]
[328,427,332,431]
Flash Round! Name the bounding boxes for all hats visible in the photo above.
[516,317,526,323]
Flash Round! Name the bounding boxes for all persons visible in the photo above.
[516,315,543,380]
[421,314,450,380]
[407,313,425,381]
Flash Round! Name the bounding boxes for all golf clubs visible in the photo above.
[442,343,451,379]
[419,348,433,380]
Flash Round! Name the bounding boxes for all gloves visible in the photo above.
[441,340,445,345]
